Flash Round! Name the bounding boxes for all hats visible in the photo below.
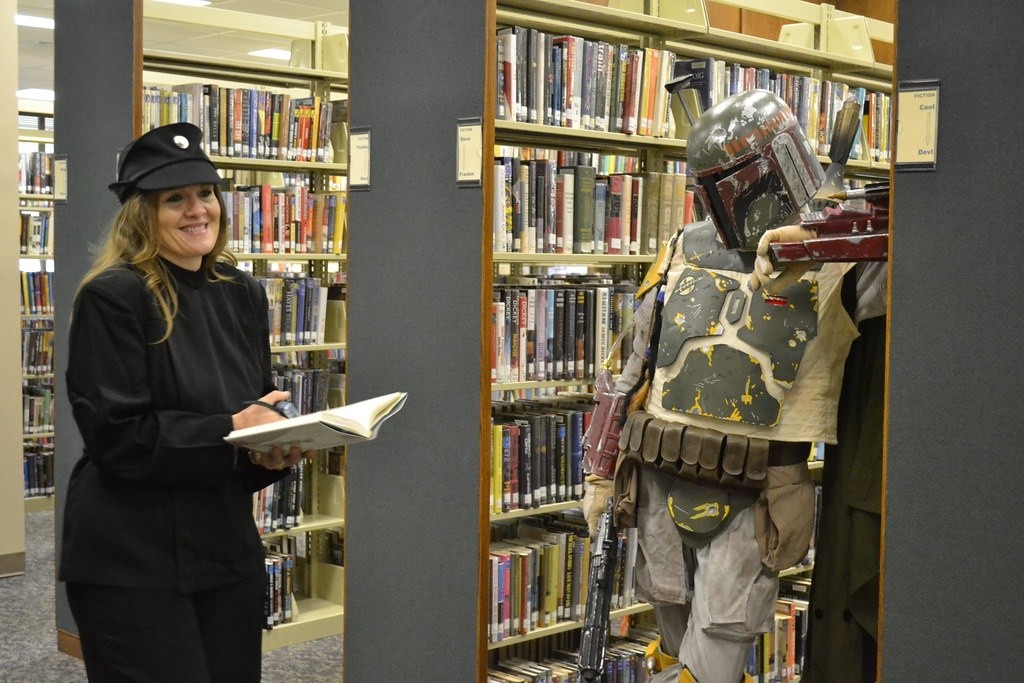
[109,122,221,204]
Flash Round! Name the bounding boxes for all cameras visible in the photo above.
[274,400,301,420]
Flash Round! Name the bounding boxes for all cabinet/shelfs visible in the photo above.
[343,0,1024,683]
[18,128,55,515]
[54,0,347,664]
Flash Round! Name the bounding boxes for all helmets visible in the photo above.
[687,88,826,254]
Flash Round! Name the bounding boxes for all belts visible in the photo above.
[768,441,812,467]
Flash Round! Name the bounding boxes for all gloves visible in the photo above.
[580,473,615,536]
[747,224,818,296]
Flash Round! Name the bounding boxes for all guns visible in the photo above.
[578,497,616,683]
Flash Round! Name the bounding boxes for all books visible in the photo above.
[223,391,409,456]
[486,27,885,683]
[139,81,347,631]
[19,149,57,497]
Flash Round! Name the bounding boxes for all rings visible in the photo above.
[272,463,284,468]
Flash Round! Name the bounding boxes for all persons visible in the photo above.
[579,89,891,682]
[58,123,317,682]
